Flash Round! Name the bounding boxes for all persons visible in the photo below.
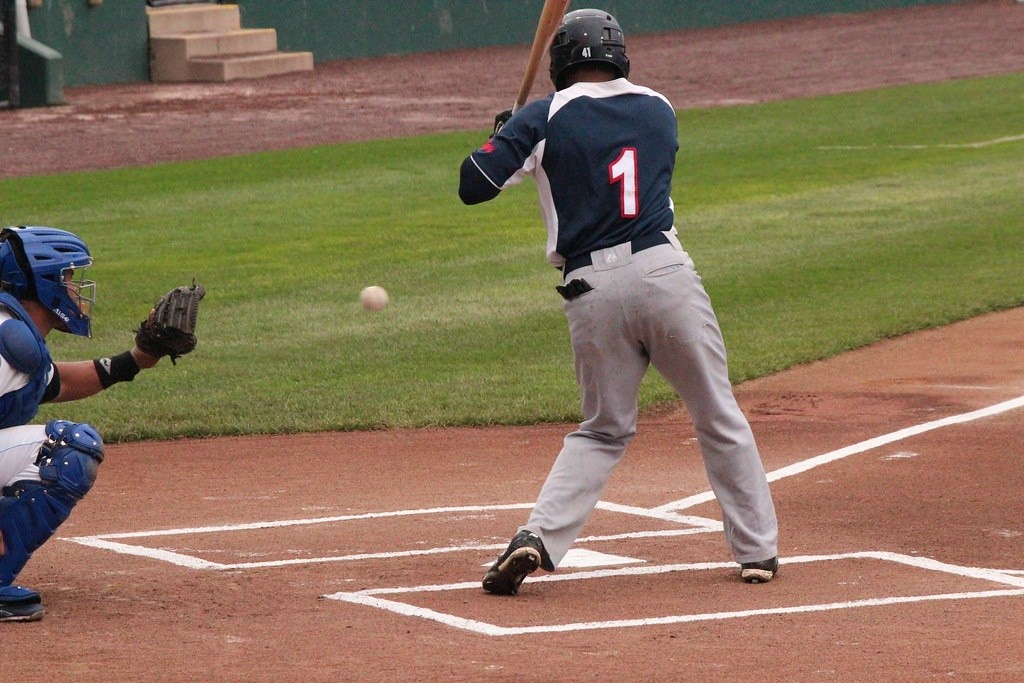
[0,224,207,621]
[458,9,778,594]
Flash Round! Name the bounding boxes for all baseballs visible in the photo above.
[360,286,390,311]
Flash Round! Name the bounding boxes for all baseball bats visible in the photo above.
[512,0,571,117]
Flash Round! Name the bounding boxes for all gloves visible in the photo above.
[555,279,591,303]
[492,109,514,136]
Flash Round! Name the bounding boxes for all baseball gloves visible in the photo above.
[134,285,206,358]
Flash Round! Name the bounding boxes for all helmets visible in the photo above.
[0,226,97,338]
[550,7,630,89]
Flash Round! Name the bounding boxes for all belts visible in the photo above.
[562,233,670,280]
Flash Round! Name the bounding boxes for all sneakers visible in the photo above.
[481,530,540,594]
[0,601,46,622]
[740,557,779,584]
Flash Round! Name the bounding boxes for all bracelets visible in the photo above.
[93,351,140,389]
